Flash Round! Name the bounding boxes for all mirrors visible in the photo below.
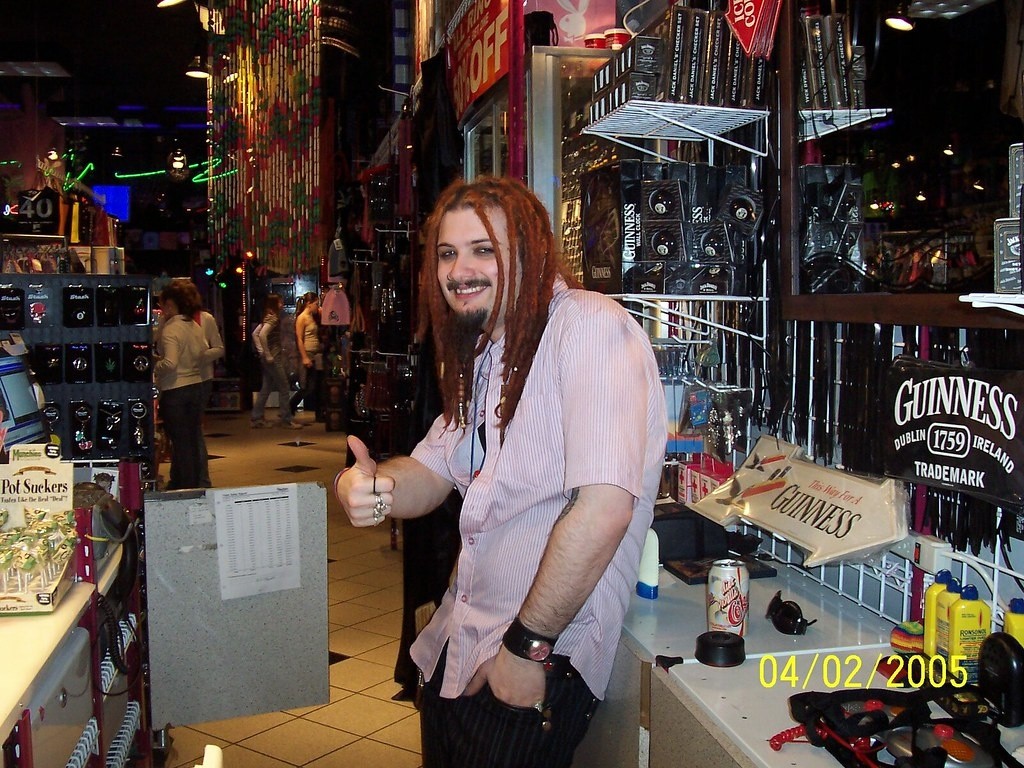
[780,0,1024,329]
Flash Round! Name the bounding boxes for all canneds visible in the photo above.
[706,560,750,641]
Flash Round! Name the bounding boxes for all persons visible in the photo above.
[333,174,669,766]
[250,292,320,429]
[153,279,226,491]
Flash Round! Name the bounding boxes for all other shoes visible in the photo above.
[249,418,275,428]
[281,420,302,430]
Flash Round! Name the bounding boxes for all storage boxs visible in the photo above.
[798,15,867,109]
[993,143,1024,293]
[589,1,771,125]
[576,158,771,296]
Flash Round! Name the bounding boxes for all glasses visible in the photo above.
[766,590,818,636]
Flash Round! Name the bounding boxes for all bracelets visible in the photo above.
[333,467,349,510]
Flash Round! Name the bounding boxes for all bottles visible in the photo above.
[948,584,990,683]
[924,569,952,657]
[1003,597,1024,649]
[935,579,962,666]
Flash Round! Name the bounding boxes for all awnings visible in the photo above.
[578,101,770,161]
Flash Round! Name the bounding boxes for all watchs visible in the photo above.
[503,617,557,663]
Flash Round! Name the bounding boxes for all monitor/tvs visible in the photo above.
[0,346,51,460]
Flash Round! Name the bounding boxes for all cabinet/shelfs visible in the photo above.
[0,481,330,768]
[579,99,771,422]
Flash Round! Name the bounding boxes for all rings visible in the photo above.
[373,493,387,528]
[372,476,377,494]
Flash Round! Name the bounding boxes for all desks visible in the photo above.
[571,556,919,768]
